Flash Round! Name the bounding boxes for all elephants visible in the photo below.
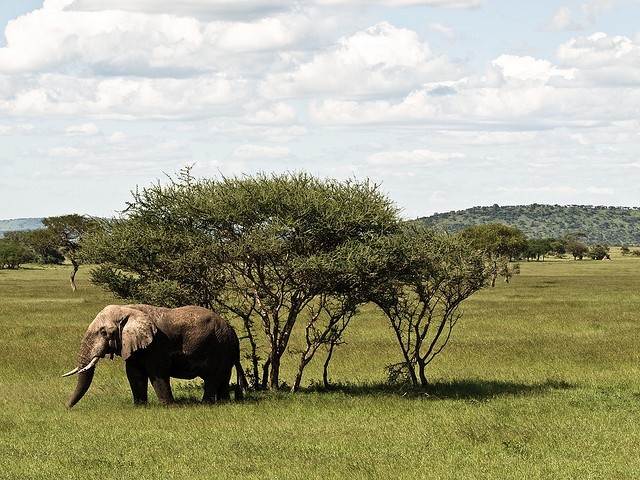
[61,303,240,412]
